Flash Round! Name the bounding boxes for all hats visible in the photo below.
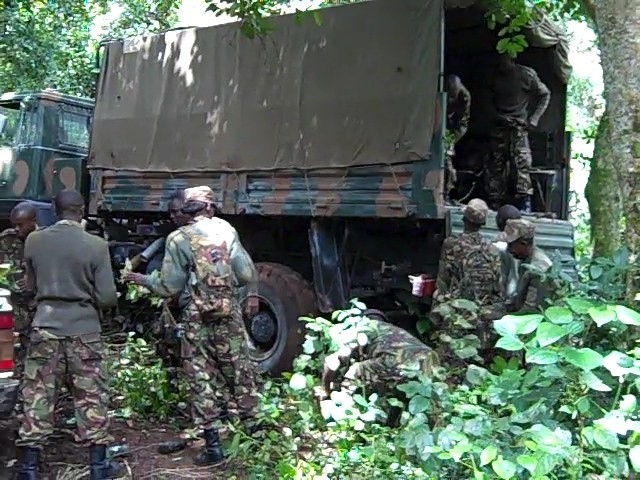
[184,186,220,209]
[461,198,488,223]
[498,219,535,243]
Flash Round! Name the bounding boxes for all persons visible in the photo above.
[17,191,123,479]
[0,202,49,382]
[495,203,549,262]
[124,184,261,463]
[444,74,473,205]
[321,307,439,400]
[432,197,507,384]
[165,194,186,227]
[484,43,560,214]
[502,219,551,314]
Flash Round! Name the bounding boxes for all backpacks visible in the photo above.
[179,226,235,321]
[453,234,496,305]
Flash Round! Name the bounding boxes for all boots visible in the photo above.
[520,196,533,217]
[90,445,121,480]
[193,429,223,466]
[16,448,39,480]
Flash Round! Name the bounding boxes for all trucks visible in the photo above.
[0,0,578,378]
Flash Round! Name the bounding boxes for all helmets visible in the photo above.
[496,205,521,224]
